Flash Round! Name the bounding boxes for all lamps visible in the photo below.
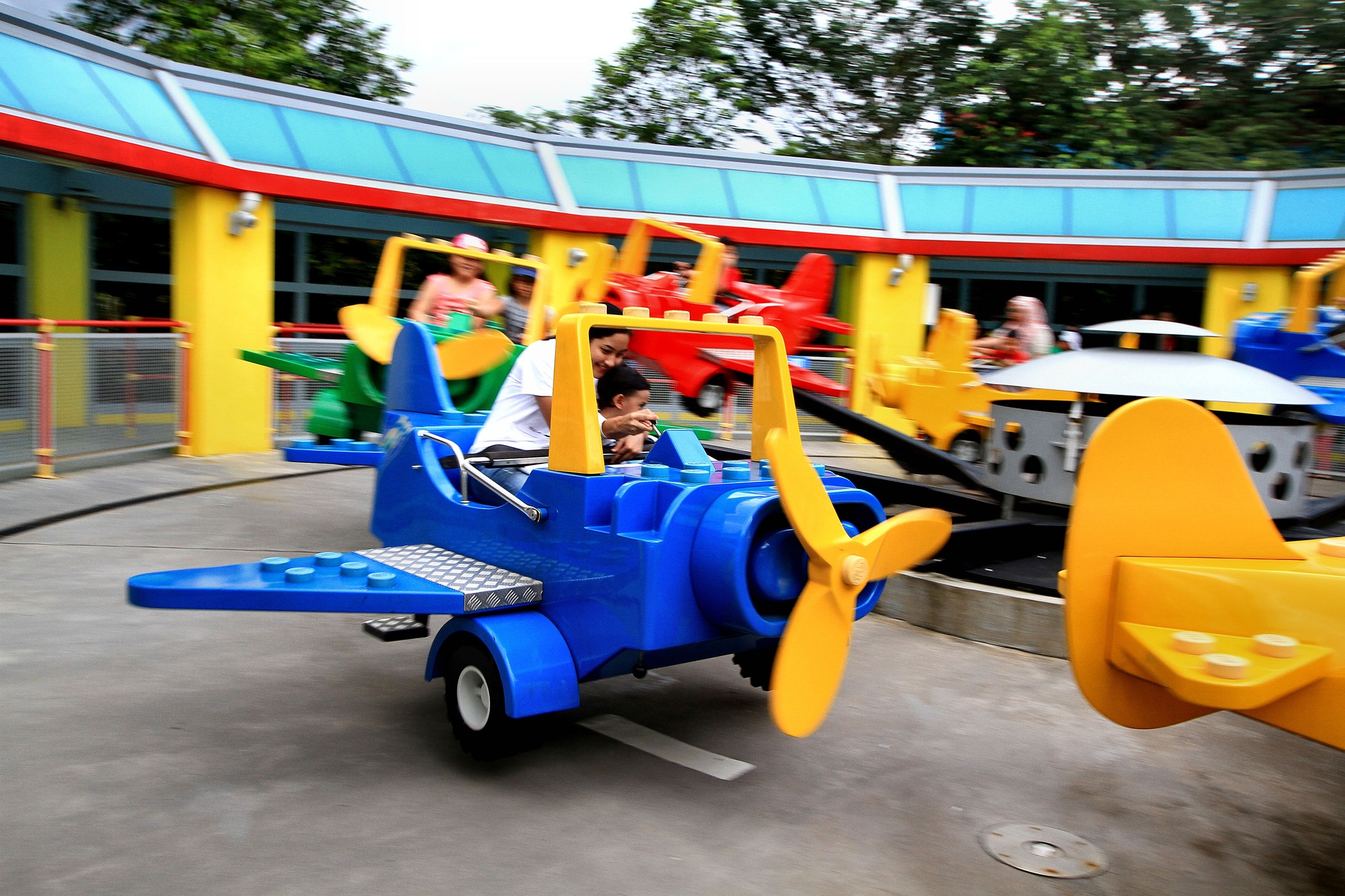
[227,191,264,235]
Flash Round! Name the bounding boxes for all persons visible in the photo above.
[676,236,744,306]
[959,292,1180,376]
[596,364,658,462]
[466,300,659,504]
[408,233,497,327]
[468,265,538,345]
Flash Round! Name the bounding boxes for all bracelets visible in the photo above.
[600,419,608,440]
